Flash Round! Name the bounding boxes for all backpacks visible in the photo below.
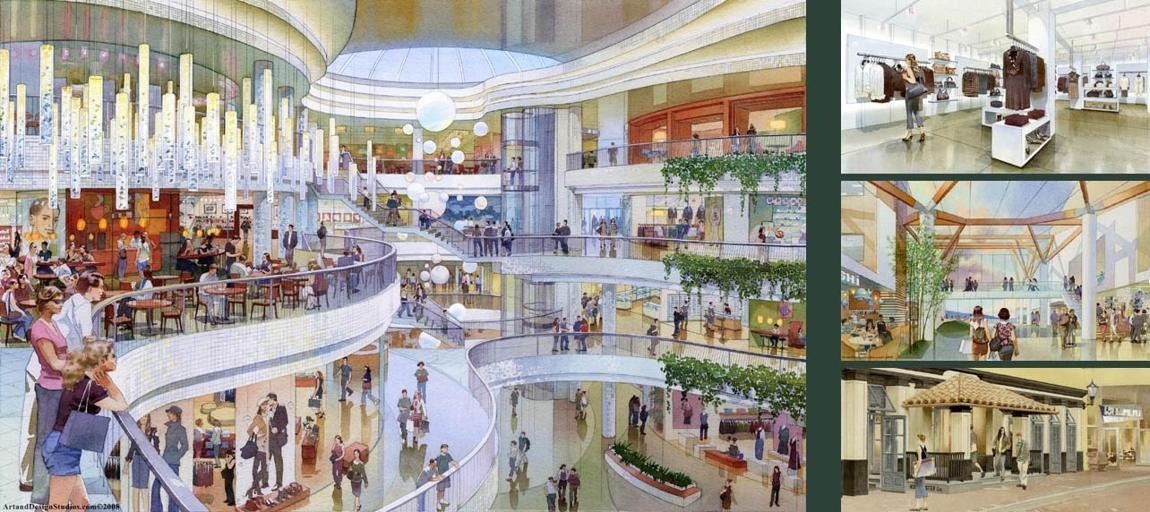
[316,226,326,240]
[522,437,531,452]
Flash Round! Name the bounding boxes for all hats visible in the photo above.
[165,405,183,417]
[225,449,234,457]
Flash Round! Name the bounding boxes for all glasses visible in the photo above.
[50,299,66,304]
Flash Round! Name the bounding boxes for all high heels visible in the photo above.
[902,134,914,143]
[918,133,926,142]
[1025,129,1049,155]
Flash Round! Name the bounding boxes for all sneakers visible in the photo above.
[548,496,577,512]
[210,316,229,326]
[1101,337,1144,344]
[333,482,342,490]
[1061,342,1076,352]
[1015,484,1027,491]
[245,482,302,512]
[223,499,235,506]
[512,412,518,417]
[574,411,588,420]
[552,347,587,354]
[353,504,362,512]
[436,498,450,511]
[505,462,529,481]
[12,331,27,343]
[337,390,381,407]
[629,422,647,435]
[769,502,781,507]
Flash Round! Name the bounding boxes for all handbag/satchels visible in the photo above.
[405,415,415,433]
[912,444,937,479]
[989,323,1002,352]
[420,417,430,433]
[944,76,957,88]
[1095,61,1113,79]
[905,66,929,100]
[972,319,989,345]
[308,396,320,408]
[240,431,259,460]
[7,290,22,322]
[58,379,113,454]
[936,88,950,101]
